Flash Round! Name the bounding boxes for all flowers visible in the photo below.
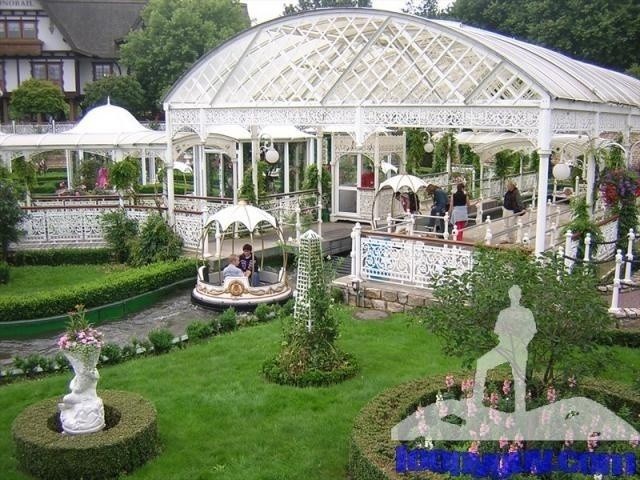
[411,376,637,478]
[60,305,101,351]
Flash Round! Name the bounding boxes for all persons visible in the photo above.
[223,254,244,281]
[236,244,260,287]
[407,187,420,230]
[394,192,411,229]
[362,166,374,187]
[424,184,450,239]
[447,183,470,241]
[460,285,537,415]
[500,179,526,244]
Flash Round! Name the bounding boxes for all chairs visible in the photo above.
[224,275,249,289]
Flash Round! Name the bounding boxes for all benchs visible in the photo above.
[209,271,277,286]
